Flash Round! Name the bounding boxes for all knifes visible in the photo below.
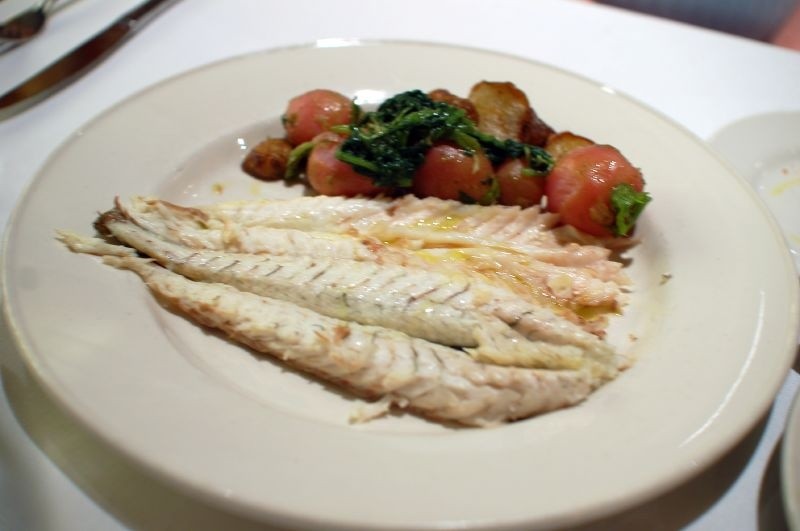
[1,0,180,124]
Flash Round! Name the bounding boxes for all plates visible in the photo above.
[1,39,799,531]
[702,109,800,278]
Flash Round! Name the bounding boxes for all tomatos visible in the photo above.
[413,142,497,201]
[280,90,358,144]
[305,134,386,195]
[543,145,645,236]
[495,155,544,207]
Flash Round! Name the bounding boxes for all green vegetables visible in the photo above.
[281,89,649,237]
